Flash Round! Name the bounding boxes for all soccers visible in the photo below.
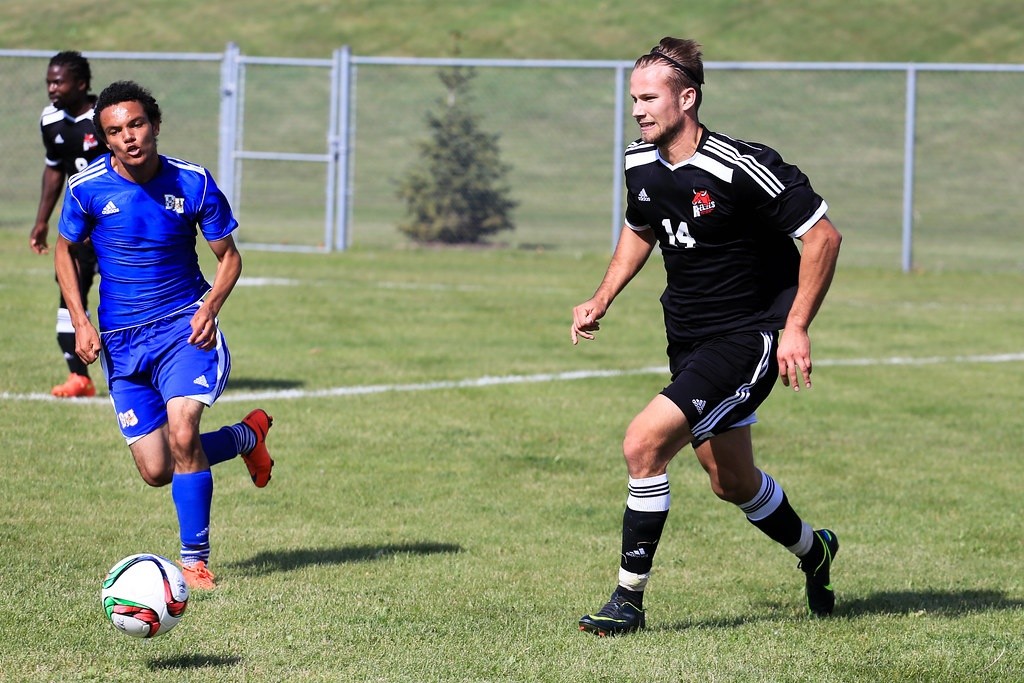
[98,553,189,640]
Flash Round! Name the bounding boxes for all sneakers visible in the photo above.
[580,590,646,634]
[180,561,215,593]
[48,373,96,399]
[796,530,839,617]
[243,408,275,487]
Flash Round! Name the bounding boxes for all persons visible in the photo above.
[569,37,843,636]
[53,81,273,591]
[28,53,117,399]
